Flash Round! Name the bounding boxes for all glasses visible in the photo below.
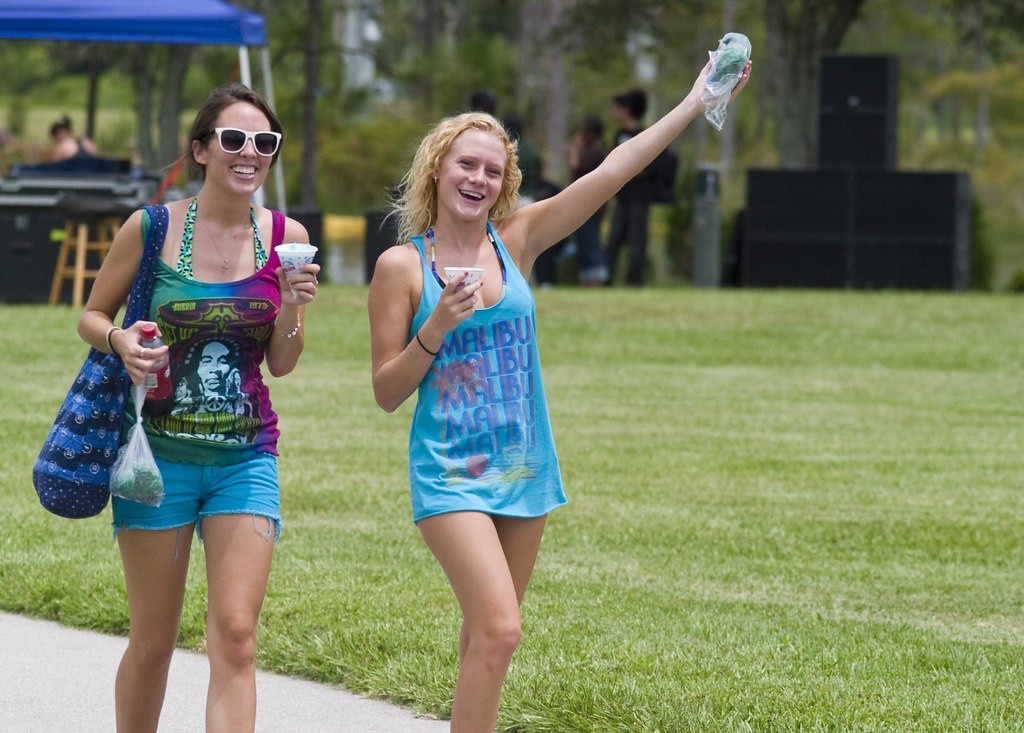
[202,127,282,156]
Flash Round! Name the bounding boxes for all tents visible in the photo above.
[1,1,288,218]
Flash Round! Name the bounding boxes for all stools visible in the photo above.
[48,197,125,311]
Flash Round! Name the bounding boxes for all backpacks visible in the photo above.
[615,125,679,186]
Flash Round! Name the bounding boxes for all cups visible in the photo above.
[443,266,486,312]
[273,242,318,300]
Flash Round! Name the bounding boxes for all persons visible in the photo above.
[468,88,660,283]
[366,50,753,730]
[79,85,319,730]
[49,116,98,164]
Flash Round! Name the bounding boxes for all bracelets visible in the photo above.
[416,330,438,355]
[273,307,301,338]
[105,326,121,354]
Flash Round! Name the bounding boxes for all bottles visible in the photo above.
[137,323,175,419]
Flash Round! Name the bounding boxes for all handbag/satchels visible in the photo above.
[33,205,171,519]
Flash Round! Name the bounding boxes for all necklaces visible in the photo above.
[194,196,249,274]
